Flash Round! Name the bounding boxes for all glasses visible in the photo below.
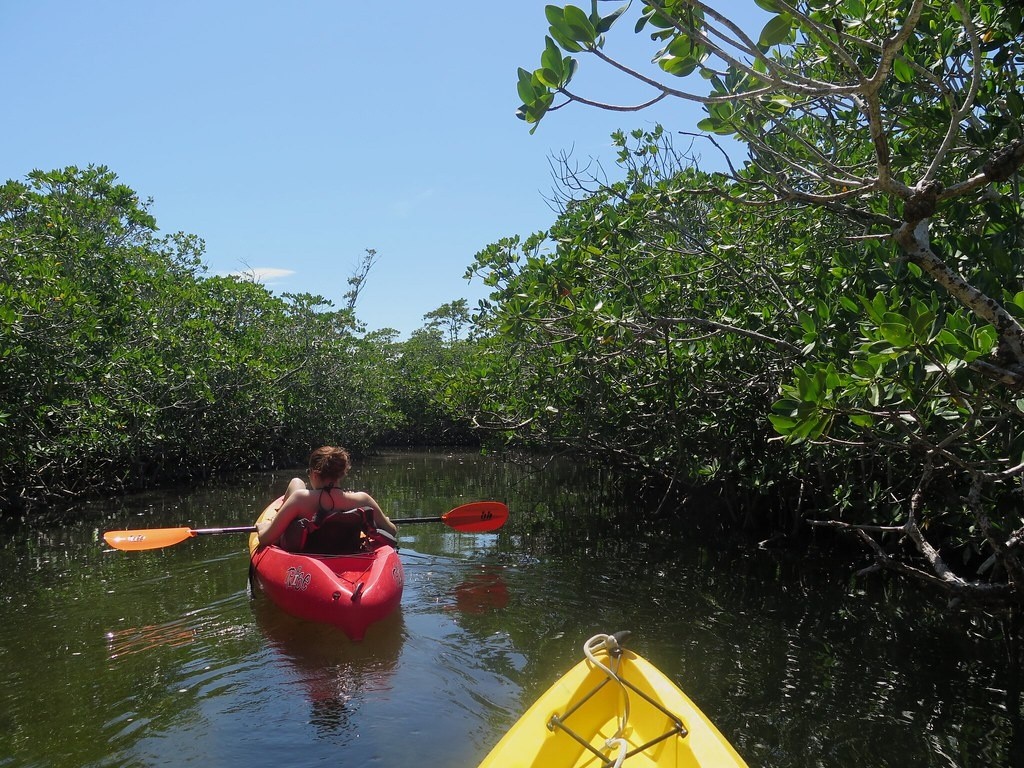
[306,469,311,477]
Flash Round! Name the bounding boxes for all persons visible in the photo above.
[256,446,397,555]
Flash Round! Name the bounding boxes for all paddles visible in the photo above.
[104,501,510,551]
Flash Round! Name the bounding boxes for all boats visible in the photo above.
[249,495,405,642]
[478,634,750,768]
